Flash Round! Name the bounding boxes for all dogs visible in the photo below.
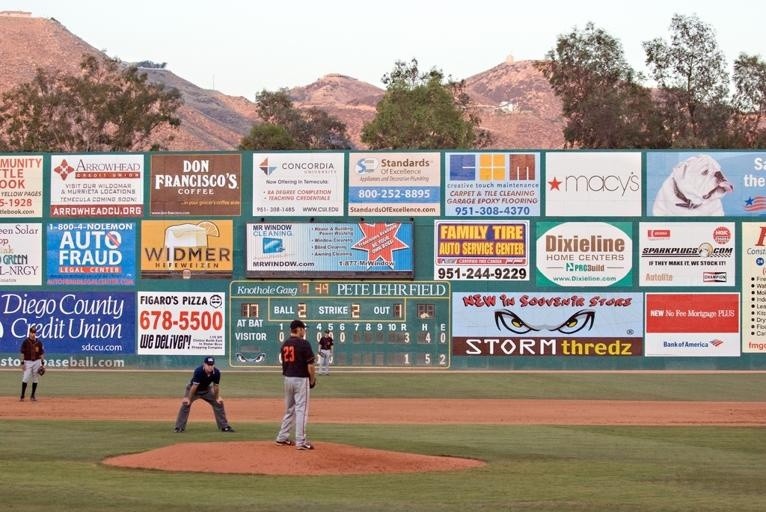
[651,155,733,216]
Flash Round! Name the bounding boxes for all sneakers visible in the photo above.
[275,438,314,450]
[20,396,36,401]
[221,426,234,432]
[174,428,183,433]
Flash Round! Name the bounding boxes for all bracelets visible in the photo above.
[21,362,24,364]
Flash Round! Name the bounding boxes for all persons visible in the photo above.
[18,326,45,402]
[175,357,233,432]
[275,320,316,449]
[318,329,333,375]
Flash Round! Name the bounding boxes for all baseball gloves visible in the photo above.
[37,366,45,375]
[320,352,325,358]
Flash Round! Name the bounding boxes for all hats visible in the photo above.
[204,357,215,364]
[30,327,36,333]
[291,320,305,329]
